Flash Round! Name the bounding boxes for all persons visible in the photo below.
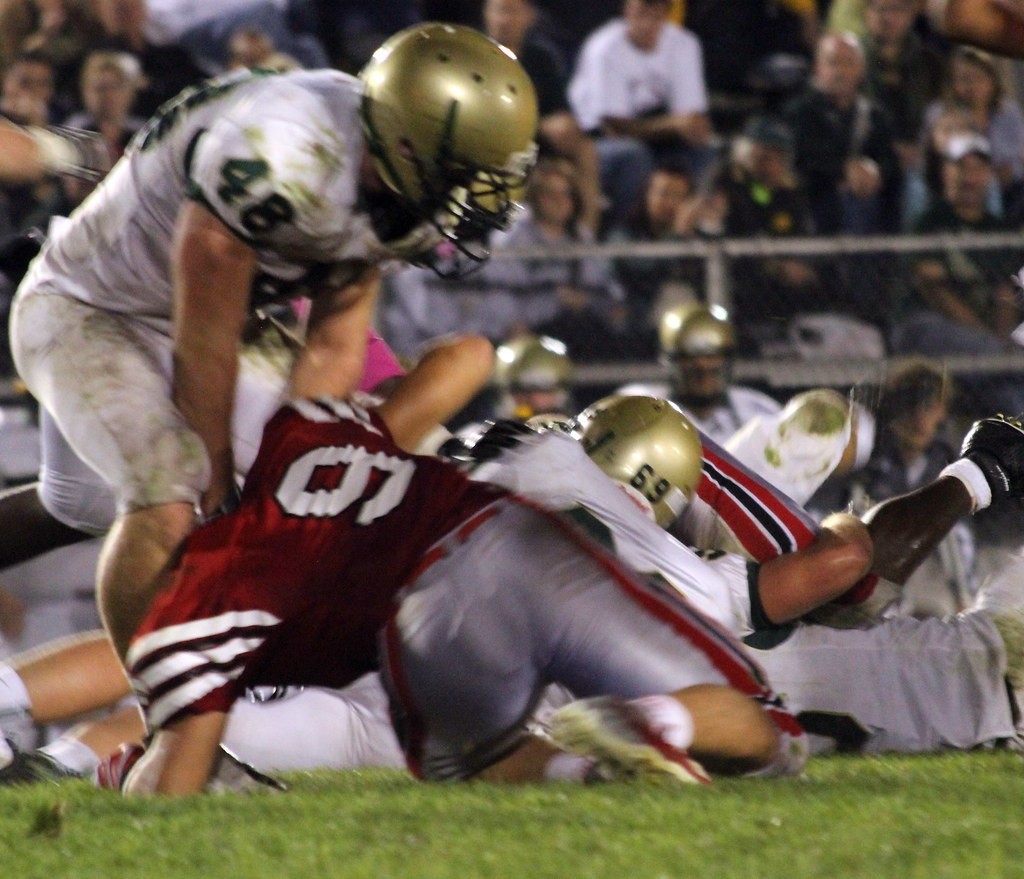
[10,24,537,792]
[2,0,1024,359]
[97,329,807,786]
[294,299,406,399]
[0,391,1024,779]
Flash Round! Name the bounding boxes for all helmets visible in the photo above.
[566,397,704,532]
[495,334,568,393]
[360,23,537,276]
[662,302,732,366]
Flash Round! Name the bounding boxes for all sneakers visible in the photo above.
[960,413,1024,491]
[543,693,714,789]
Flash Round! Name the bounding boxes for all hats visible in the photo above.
[950,132,993,162]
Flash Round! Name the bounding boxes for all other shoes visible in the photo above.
[6,738,65,791]
